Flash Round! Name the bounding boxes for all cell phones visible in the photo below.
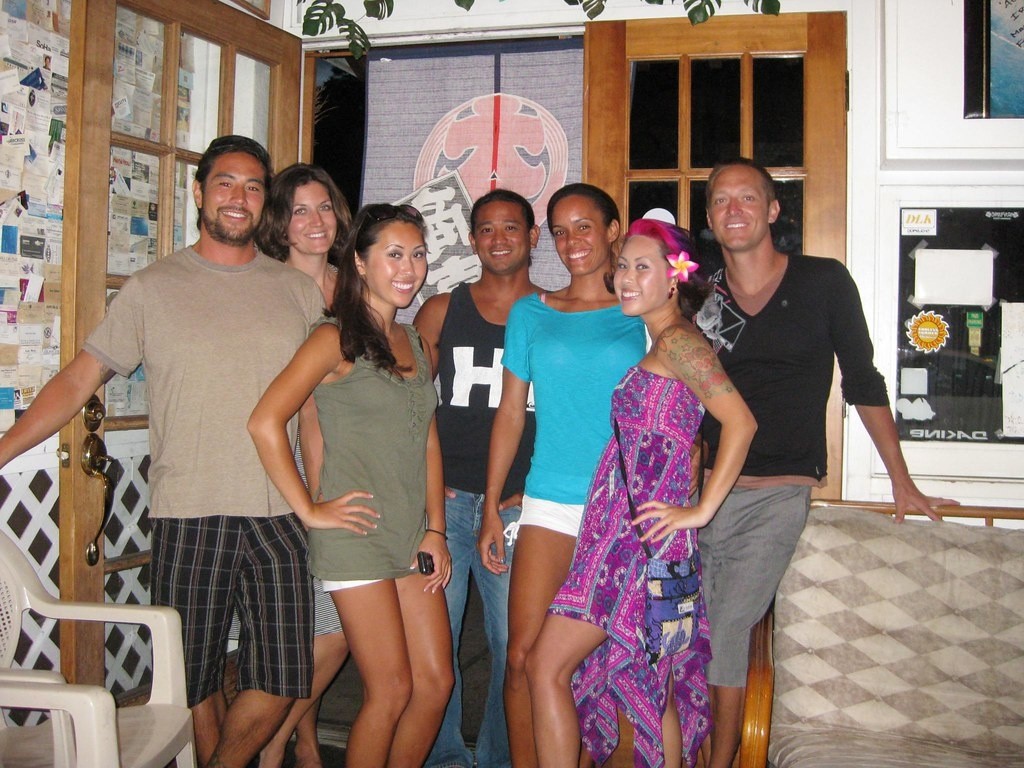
[417,552,435,575]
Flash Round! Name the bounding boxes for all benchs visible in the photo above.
[739,499,1024,768]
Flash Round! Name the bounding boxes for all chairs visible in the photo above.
[0,530,198,768]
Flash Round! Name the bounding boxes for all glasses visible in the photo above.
[199,135,271,170]
[360,205,423,230]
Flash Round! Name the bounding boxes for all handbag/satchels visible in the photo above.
[645,550,700,665]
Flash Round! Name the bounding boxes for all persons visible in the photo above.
[0,135,457,768]
[478,157,960,768]
[412,188,556,768]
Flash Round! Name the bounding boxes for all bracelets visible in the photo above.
[426,529,448,540]
